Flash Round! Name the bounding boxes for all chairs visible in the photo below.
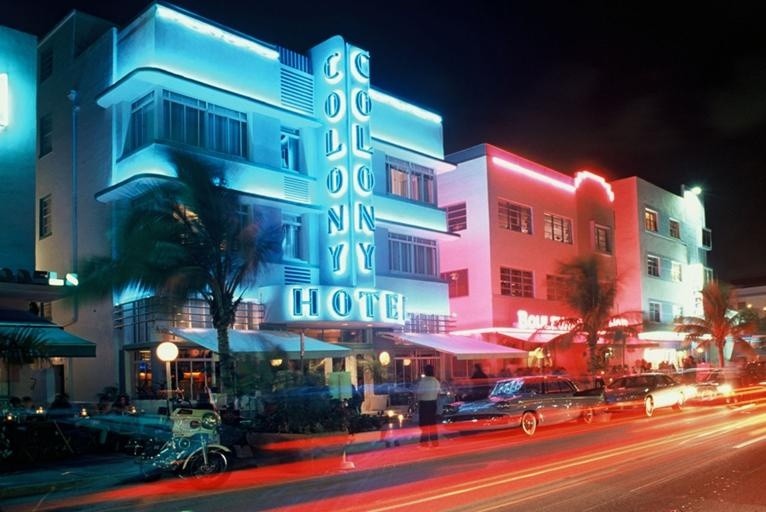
[0,406,167,469]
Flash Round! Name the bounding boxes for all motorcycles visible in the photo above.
[126,414,232,491]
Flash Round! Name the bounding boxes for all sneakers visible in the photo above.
[420,442,439,446]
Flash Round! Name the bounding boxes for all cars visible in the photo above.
[358,360,766,437]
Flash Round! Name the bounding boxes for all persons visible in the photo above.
[3,397,23,451]
[111,392,137,417]
[498,356,697,380]
[173,383,184,401]
[415,363,440,448]
[347,385,362,416]
[195,386,218,413]
[470,362,490,400]
[47,392,72,437]
[96,398,120,417]
[22,397,35,422]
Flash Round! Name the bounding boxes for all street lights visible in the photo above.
[156,341,180,416]
